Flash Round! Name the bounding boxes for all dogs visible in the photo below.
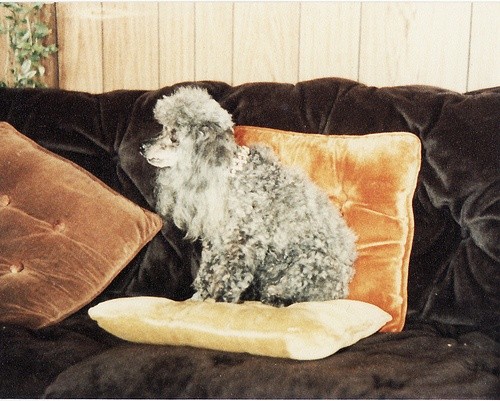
[138,84,358,309]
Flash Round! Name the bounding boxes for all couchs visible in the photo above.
[0,77,500,401]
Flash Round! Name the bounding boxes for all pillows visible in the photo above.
[233,123,424,336]
[88,294,394,362]
[0,119,167,335]
